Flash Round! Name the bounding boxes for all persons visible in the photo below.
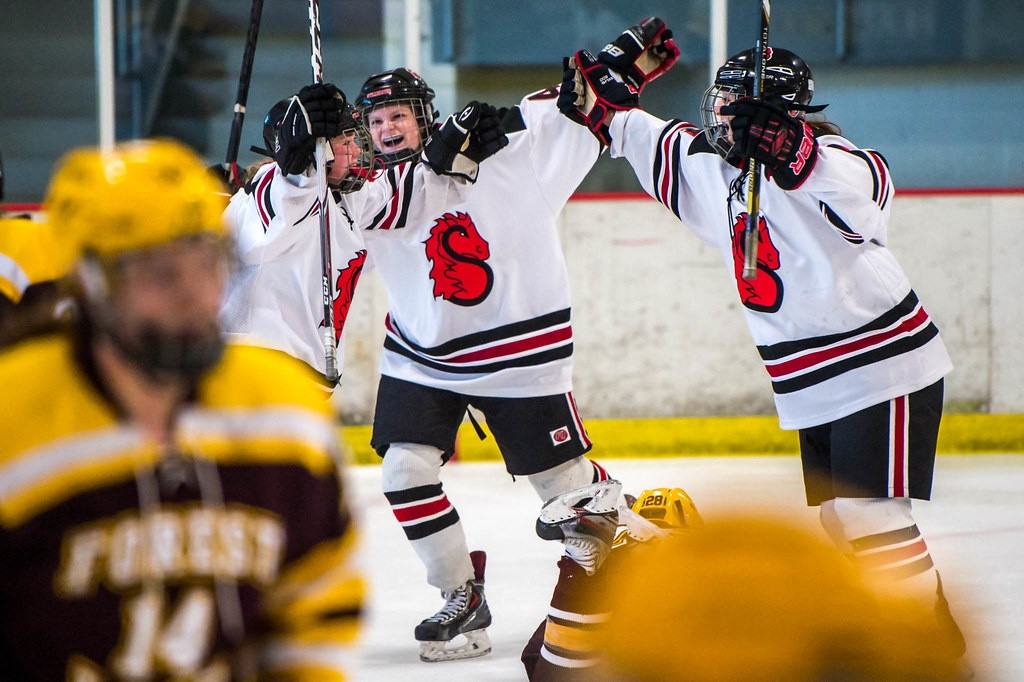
[0,135,983,682]
[206,14,683,663]
[557,46,979,677]
[215,81,509,373]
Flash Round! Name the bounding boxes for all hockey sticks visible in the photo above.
[741,0,769,279]
[304,1,340,383]
[223,0,266,184]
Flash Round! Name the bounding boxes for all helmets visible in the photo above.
[249,93,375,194]
[352,67,435,165]
[47,137,229,272]
[701,48,830,170]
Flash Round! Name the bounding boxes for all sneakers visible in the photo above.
[414,548,492,661]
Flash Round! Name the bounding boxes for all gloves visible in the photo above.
[578,15,680,110]
[556,49,638,145]
[274,83,352,173]
[718,97,818,190]
[421,100,510,183]
